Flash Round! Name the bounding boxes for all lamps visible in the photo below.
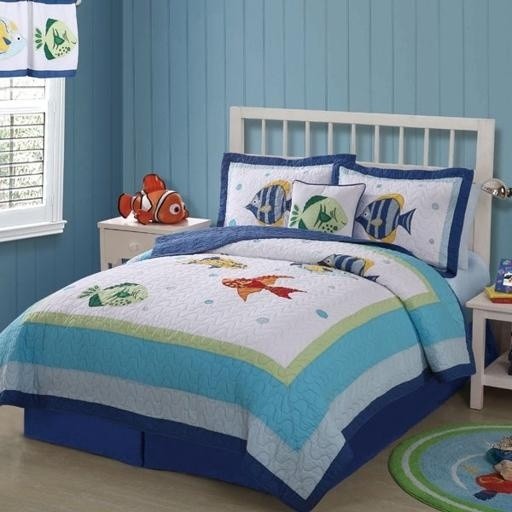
[481,178,512,201]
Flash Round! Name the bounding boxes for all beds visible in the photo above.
[0,105,498,512]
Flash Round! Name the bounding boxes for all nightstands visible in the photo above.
[98,216,210,271]
[466,284,512,411]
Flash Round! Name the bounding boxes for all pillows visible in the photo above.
[330,158,480,279]
[216,152,358,230]
[289,181,365,238]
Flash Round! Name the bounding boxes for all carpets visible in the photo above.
[388,420,512,512]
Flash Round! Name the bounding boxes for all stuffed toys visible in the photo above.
[117,174,188,224]
